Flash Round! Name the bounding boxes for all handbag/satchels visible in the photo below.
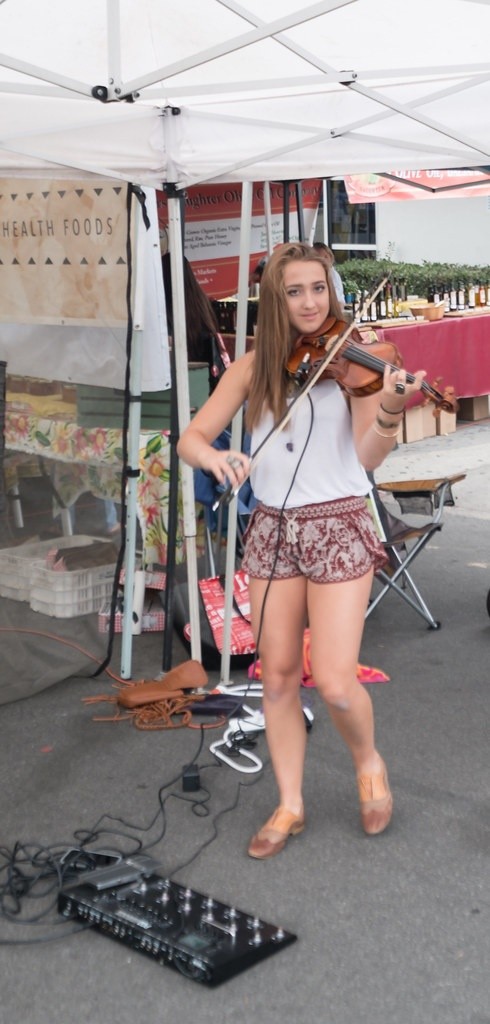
[157,564,260,671]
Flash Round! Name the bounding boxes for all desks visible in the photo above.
[220,315,490,409]
[4,392,170,635]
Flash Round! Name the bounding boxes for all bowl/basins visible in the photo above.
[410,303,445,320]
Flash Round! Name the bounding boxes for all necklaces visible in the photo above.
[283,400,299,452]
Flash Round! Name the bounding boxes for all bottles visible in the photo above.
[353,276,490,323]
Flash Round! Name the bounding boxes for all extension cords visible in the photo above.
[227,706,314,736]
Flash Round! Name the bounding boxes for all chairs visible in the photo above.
[362,464,467,630]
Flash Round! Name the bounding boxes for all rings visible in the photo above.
[226,454,241,468]
[394,383,406,394]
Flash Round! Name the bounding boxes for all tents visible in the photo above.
[0,0,490,690]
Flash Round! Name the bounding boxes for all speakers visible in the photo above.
[171,582,259,674]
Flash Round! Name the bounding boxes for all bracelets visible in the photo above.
[379,402,405,414]
[376,416,399,428]
[372,424,399,437]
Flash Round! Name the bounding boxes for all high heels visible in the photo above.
[357,755,394,835]
[248,802,305,859]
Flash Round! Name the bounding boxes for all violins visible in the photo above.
[280,313,460,416]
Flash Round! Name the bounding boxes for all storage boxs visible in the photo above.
[98,570,166,631]
[399,394,490,445]
[1,534,117,618]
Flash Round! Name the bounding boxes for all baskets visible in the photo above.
[409,302,444,320]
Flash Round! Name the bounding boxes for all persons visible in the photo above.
[313,242,347,314]
[176,244,426,859]
[160,251,230,378]
